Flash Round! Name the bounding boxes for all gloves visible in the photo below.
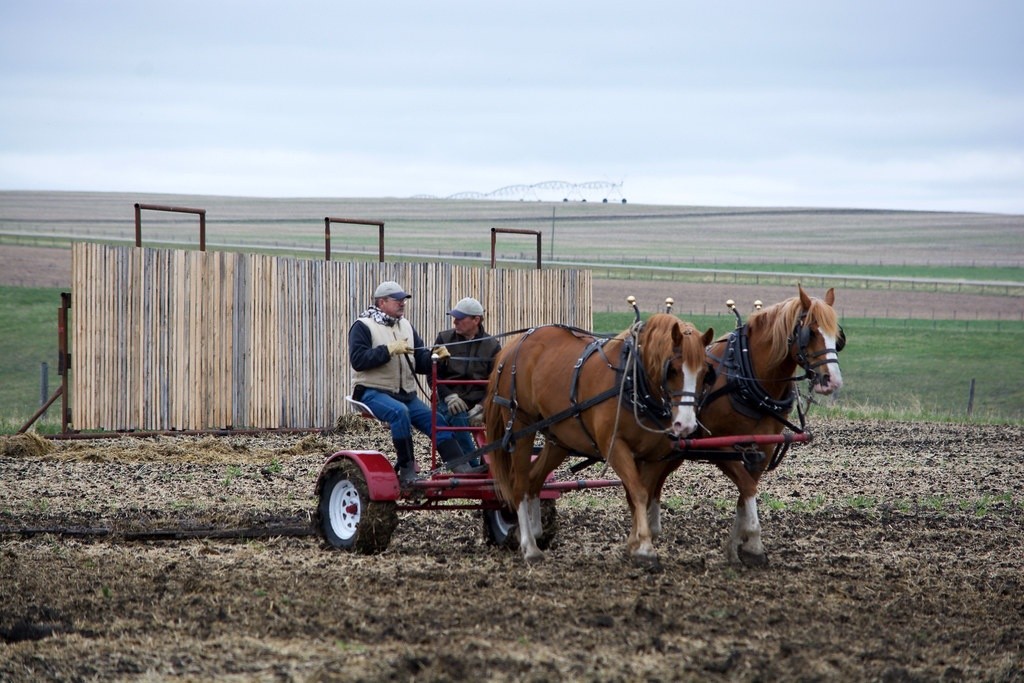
[432,345,450,363]
[468,404,485,429]
[387,338,415,357]
[445,392,469,415]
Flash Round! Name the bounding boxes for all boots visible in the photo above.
[393,435,424,482]
[435,436,487,476]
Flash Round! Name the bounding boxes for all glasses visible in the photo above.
[389,298,408,303]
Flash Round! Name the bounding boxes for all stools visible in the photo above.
[345,393,422,473]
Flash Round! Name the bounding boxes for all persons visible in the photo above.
[425,297,503,468]
[345,282,489,485]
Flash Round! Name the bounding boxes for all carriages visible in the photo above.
[311,283,842,569]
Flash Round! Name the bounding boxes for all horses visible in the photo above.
[485,313,713,575]
[621,283,843,568]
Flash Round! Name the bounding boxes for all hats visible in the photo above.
[374,281,412,301]
[446,297,484,320]
